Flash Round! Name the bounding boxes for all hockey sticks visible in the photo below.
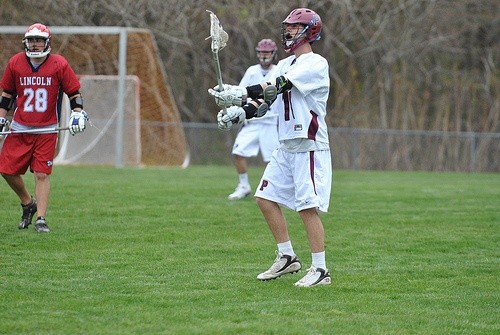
[208,13,230,115]
[0,125,87,136]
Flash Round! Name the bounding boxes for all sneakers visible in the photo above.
[257,249,302,281]
[293,266,331,287]
[18,195,37,229]
[34,216,50,233]
[229,184,251,200]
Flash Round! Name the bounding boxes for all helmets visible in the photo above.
[280,7,323,55]
[22,23,52,58]
[255,39,277,68]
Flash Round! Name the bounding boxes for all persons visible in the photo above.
[0,23,85,234]
[208,8,333,287]
[227,39,279,200]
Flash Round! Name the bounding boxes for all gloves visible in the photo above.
[207,82,248,108]
[68,110,89,136]
[0,116,10,138]
[216,104,246,131]
